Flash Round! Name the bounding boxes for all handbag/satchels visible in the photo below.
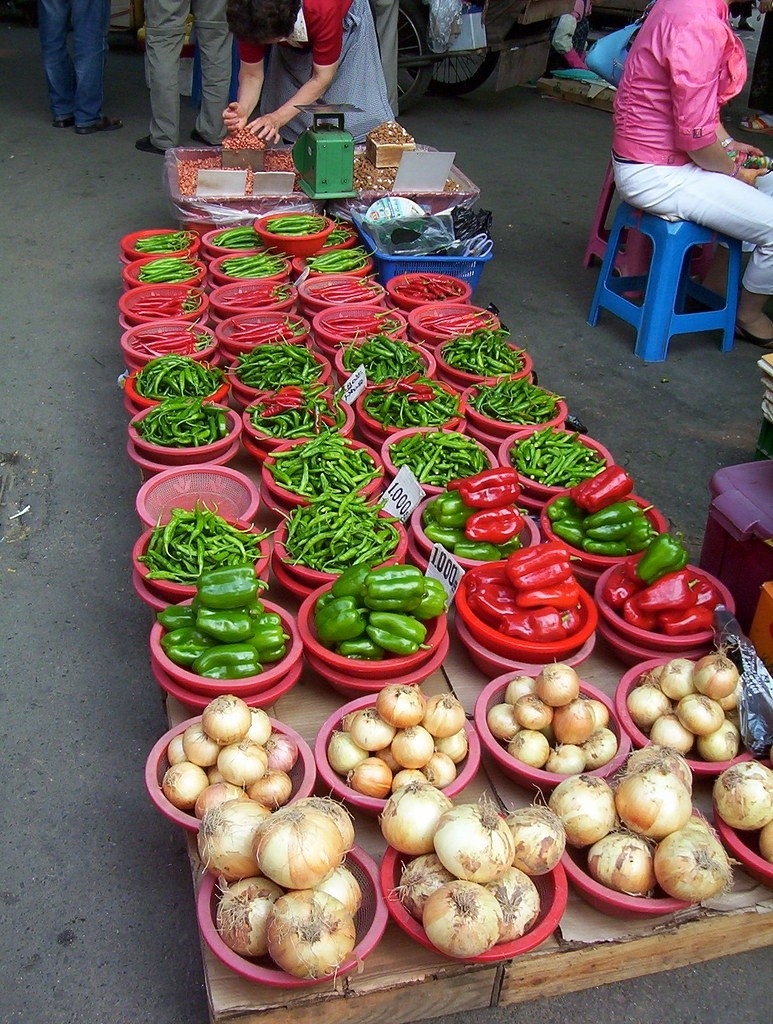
[585,0,656,88]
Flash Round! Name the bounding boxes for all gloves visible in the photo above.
[564,49,587,69]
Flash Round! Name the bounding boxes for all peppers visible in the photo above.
[135,229,204,284]
[339,330,609,489]
[424,465,728,636]
[213,213,377,277]
[131,341,400,583]
[315,562,448,661]
[155,561,291,681]
[127,286,305,357]
[308,269,498,338]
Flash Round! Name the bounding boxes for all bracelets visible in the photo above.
[721,136,733,148]
[730,163,740,178]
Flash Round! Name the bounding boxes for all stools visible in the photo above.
[586,198,740,362]
[585,157,719,297]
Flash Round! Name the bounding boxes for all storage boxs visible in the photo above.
[698,460,773,637]
[164,147,328,241]
[330,143,479,228]
[354,212,491,301]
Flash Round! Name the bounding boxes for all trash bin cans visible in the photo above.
[698,459,773,637]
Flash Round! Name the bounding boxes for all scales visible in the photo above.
[292,103,366,200]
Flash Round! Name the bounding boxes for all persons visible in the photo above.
[611,0,773,349]
[739,0,773,136]
[39,0,125,134]
[553,0,590,69]
[728,0,757,32]
[135,0,231,155]
[223,0,394,145]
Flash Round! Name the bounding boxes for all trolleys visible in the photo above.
[398,0,551,115]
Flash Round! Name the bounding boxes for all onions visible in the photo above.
[160,683,566,981]
[488,650,772,903]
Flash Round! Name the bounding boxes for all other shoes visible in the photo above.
[52,117,74,128]
[191,130,222,146]
[135,136,168,155]
[76,116,123,133]
[733,322,773,348]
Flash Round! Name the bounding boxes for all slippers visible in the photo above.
[742,114,759,122]
[739,118,773,135]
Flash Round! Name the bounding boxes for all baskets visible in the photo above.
[117,207,773,984]
